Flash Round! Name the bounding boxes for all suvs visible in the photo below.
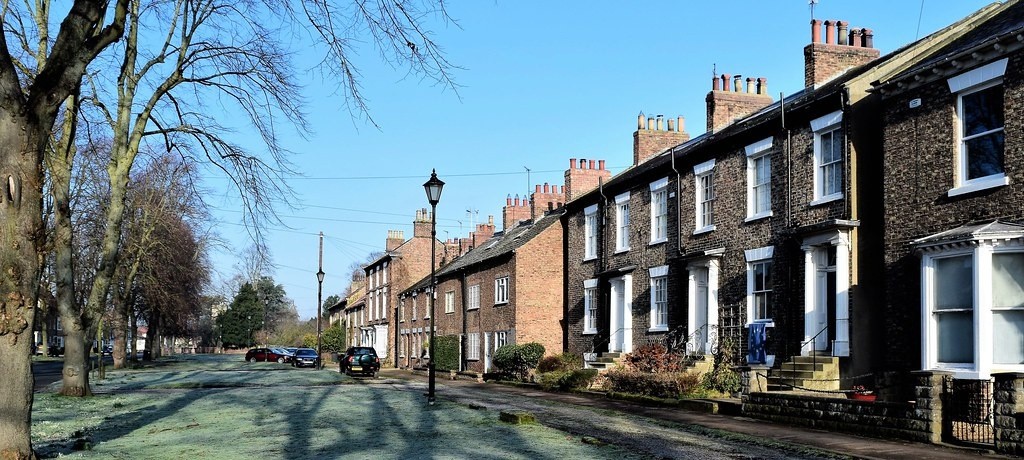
[337,346,381,378]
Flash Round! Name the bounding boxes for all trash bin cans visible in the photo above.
[845,392,877,401]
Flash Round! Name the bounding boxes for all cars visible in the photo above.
[290,348,319,368]
[244,346,298,364]
[35,341,55,356]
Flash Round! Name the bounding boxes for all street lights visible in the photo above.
[315,267,326,370]
[422,167,445,405]
[264,295,269,362]
